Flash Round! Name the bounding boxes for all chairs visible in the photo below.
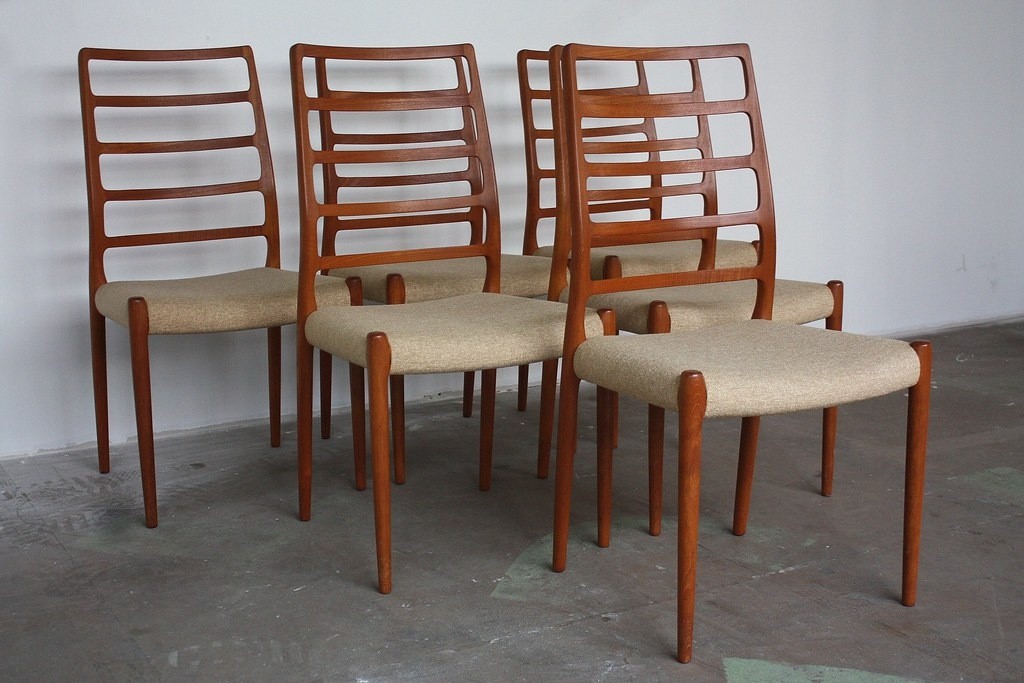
[79,45,368,532]
[536,45,843,536]
[290,42,616,595]
[320,55,574,486]
[515,48,767,411]
[549,41,932,663]
